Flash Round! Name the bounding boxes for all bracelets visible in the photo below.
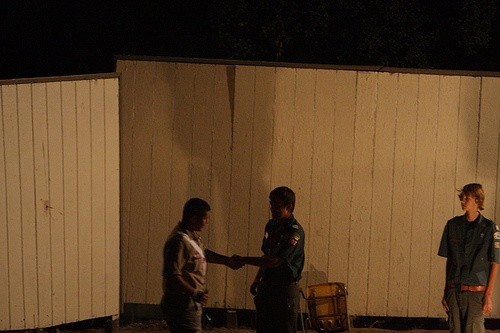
[193,289,202,298]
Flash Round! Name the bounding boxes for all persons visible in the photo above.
[438,184,500,333]
[159,197,246,333]
[227,187,305,333]
